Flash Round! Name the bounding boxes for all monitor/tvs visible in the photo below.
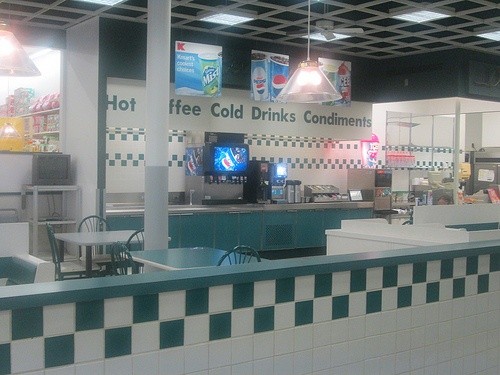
[32,153,72,185]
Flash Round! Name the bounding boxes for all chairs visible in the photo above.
[78,216,144,275]
[217,245,261,267]
[45,223,100,282]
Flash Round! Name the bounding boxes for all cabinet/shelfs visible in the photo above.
[25,187,81,263]
[15,108,59,152]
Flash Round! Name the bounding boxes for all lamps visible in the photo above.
[0,28,41,78]
[273,0,342,105]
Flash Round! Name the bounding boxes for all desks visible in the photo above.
[54,231,171,279]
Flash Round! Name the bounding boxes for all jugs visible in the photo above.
[286,179,295,203]
[295,180,302,202]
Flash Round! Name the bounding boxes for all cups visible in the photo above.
[218,52,222,94]
[198,51,218,94]
[305,197,310,203]
[321,64,338,106]
[338,71,351,107]
[184,150,200,175]
[251,53,269,101]
[301,197,305,203]
[269,55,290,104]
[214,148,239,171]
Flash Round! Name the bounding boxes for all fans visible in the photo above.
[312,1,364,42]
